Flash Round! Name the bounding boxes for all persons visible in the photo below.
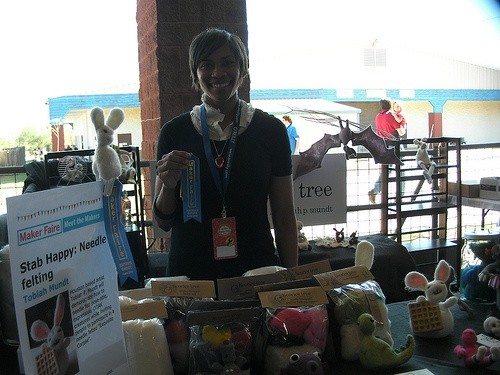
[282,115,301,155]
[368,99,406,204]
[151,28,298,282]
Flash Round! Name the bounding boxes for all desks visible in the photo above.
[387,295,500,375]
[434,190,500,230]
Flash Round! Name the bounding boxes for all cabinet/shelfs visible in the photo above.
[46,148,148,259]
[383,138,462,287]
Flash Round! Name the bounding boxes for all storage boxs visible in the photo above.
[448,177,500,200]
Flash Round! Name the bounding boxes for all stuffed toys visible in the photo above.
[62,107,136,197]
[169,117,500,375]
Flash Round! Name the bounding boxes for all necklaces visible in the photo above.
[208,127,233,168]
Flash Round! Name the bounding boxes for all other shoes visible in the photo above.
[368,190,375,203]
